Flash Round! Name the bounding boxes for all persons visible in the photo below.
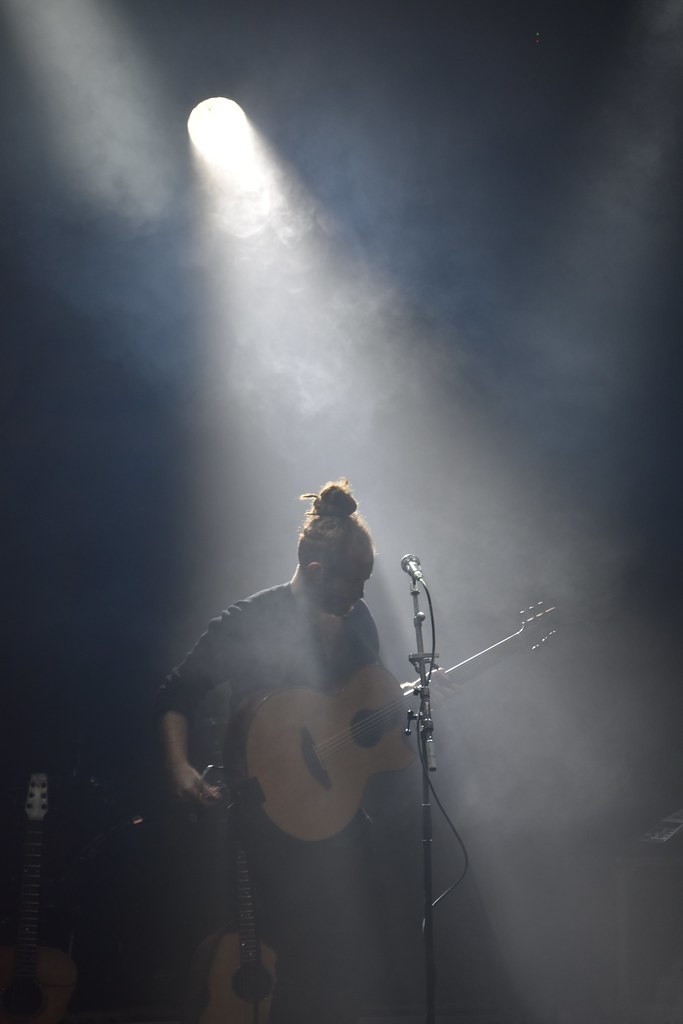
[156,485,465,1024]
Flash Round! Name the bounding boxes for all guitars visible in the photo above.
[230,597,574,851]
[1,766,82,1024]
[196,828,284,1024]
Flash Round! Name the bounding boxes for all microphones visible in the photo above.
[401,554,422,581]
